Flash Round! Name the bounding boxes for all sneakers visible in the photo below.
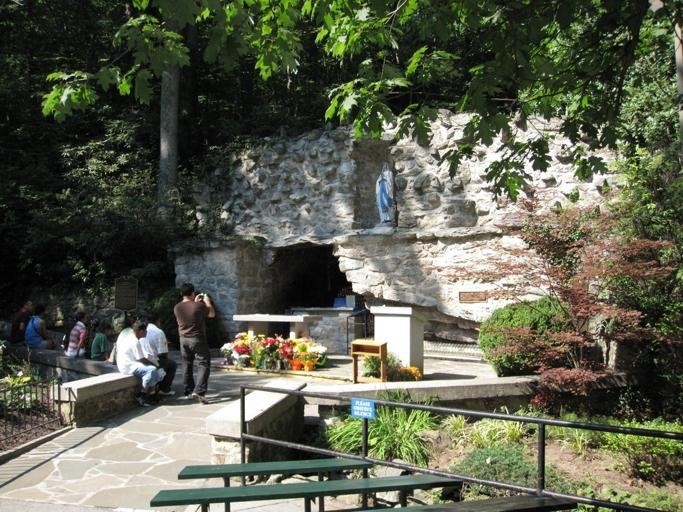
[135,390,176,407]
[185,392,208,404]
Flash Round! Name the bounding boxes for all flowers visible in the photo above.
[218,329,327,373]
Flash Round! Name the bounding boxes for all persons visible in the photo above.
[89,321,112,361]
[108,311,178,407]
[24,304,57,350]
[62,312,87,357]
[173,282,216,404]
[9,299,33,345]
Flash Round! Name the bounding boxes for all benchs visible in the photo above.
[150,459,578,512]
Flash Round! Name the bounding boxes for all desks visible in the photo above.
[349,337,388,385]
